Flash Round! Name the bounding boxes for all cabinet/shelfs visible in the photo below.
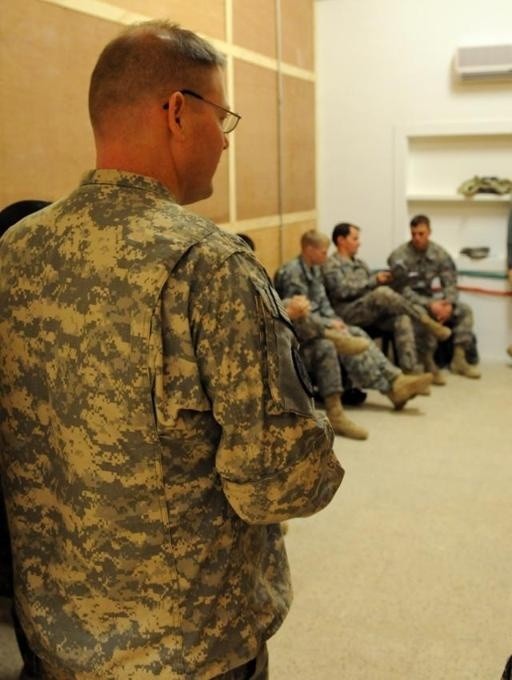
[393,125,512,298]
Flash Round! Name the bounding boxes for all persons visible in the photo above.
[387,215,480,385]
[320,223,452,395]
[507,213,512,355]
[274,230,434,410]
[237,233,370,440]
[0,20,345,680]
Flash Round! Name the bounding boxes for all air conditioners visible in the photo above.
[453,43,512,79]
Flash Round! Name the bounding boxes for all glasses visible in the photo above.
[163,91,240,132]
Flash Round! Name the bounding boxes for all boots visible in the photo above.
[450,344,481,378]
[420,314,452,341]
[325,329,369,355]
[324,393,368,440]
[384,366,446,410]
